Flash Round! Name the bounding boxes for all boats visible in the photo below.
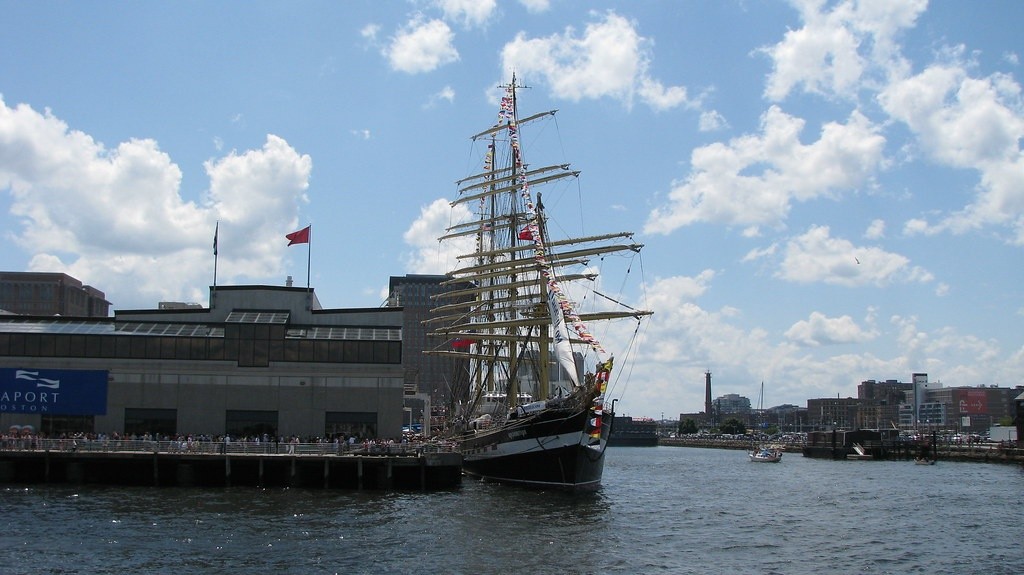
[847,453,874,461]
[746,447,784,462]
[605,413,659,447]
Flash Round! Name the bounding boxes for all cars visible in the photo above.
[673,432,808,442]
[899,432,992,443]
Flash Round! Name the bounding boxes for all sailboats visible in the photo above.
[415,68,654,493]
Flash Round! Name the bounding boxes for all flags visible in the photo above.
[474,88,607,353]
[286,225,311,247]
[452,328,477,347]
[587,357,614,452]
[213,220,218,256]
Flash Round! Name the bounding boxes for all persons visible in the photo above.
[0,430,459,456]
[752,449,778,457]
[683,435,807,443]
[960,436,984,447]
[413,415,479,429]
[1002,439,1004,444]
